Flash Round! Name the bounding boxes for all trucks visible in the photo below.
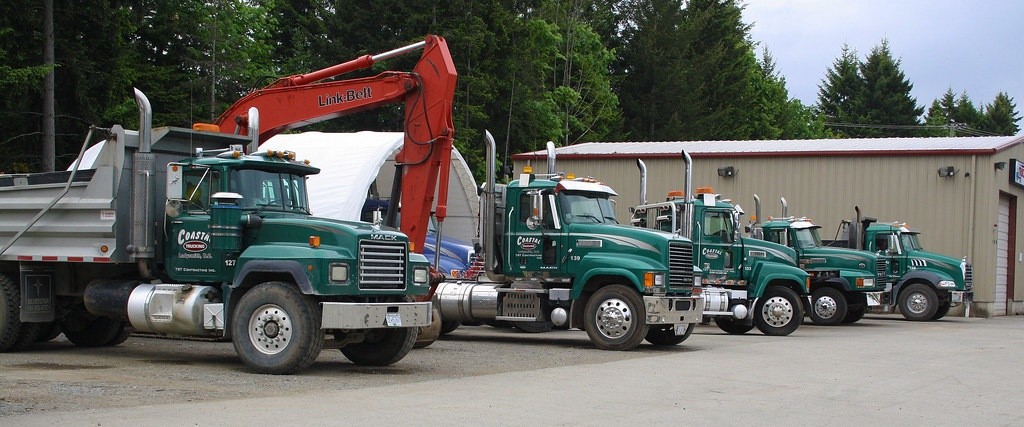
[816,205,974,322]
[737,193,888,326]
[248,131,706,353]
[0,86,434,376]
[629,146,815,335]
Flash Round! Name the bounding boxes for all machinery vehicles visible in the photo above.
[217,33,460,302]
